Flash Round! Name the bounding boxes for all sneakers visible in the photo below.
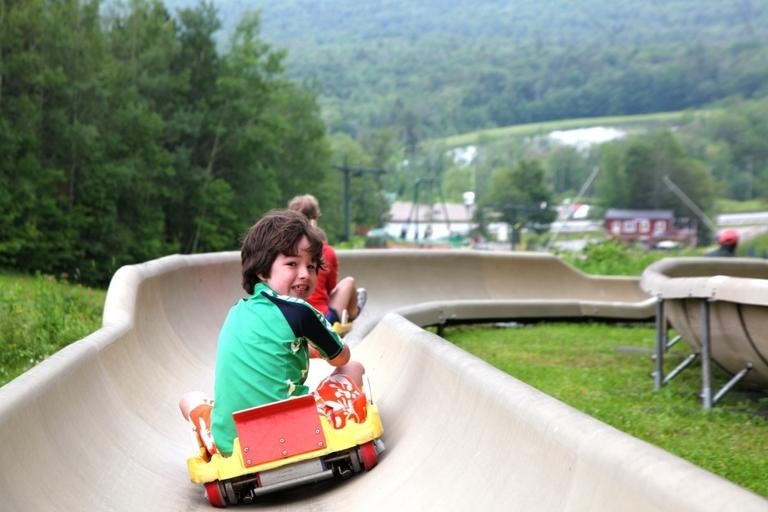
[349,288,367,322]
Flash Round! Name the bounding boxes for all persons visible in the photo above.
[703,227,742,256]
[212,208,387,461]
[285,192,369,344]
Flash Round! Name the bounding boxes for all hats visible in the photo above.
[718,229,738,245]
